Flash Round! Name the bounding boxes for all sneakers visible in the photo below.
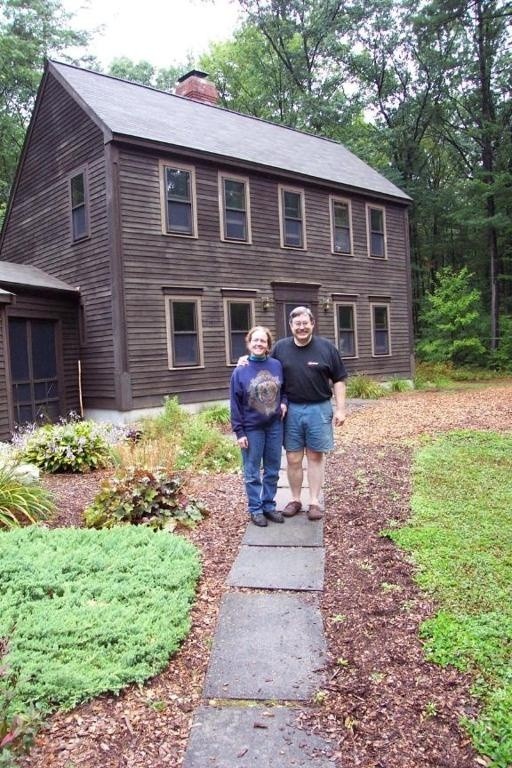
[250,512,268,527]
[306,502,324,520]
[281,500,302,517]
[263,508,284,523]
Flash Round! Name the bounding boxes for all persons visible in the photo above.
[236,306,348,520]
[229,326,289,527]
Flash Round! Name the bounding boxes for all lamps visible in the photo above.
[263,296,271,310]
[323,298,333,311]
